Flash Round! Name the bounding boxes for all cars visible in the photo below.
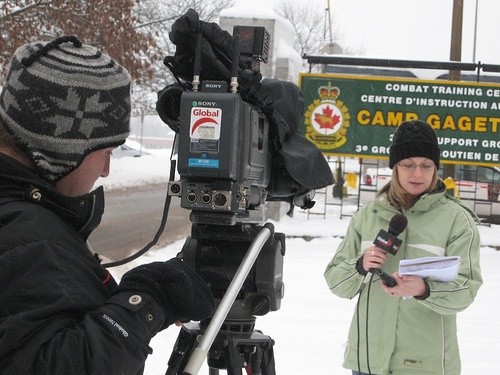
[365,162,500,216]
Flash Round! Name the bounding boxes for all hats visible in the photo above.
[389,121,442,172]
[0,36,136,184]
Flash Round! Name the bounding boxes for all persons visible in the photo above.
[323,120,482,375]
[1,35,212,375]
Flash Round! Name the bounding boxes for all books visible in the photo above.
[398,255,460,301]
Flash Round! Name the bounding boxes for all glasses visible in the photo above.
[394,157,435,171]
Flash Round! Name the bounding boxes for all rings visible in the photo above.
[371,250,374,256]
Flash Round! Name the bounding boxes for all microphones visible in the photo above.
[359,213,408,296]
[374,268,398,289]
